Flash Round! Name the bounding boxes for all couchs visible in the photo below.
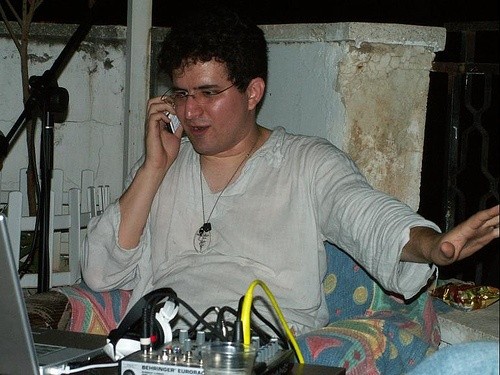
[21,235,442,375]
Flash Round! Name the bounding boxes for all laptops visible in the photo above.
[0,215,109,375]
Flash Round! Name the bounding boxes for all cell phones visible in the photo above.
[164,111,181,134]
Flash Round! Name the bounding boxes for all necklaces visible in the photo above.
[193,130,260,253]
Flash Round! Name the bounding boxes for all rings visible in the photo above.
[493,224,497,228]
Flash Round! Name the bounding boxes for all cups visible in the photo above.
[200,341,257,375]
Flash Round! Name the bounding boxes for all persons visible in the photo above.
[80,10,500,339]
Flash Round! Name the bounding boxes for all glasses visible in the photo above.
[160,81,242,107]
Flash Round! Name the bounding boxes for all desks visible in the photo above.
[32,329,347,375]
